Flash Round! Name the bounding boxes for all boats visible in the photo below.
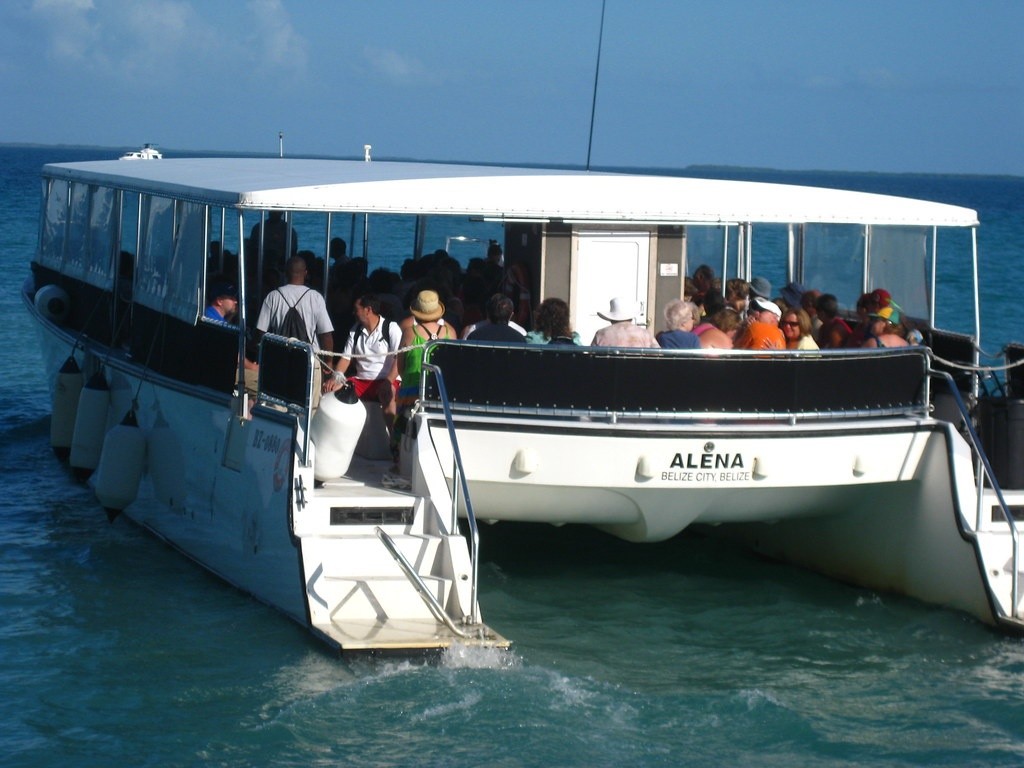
[18,133,1024,658]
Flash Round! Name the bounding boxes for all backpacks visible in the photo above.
[274,289,317,345]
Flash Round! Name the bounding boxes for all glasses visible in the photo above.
[781,320,800,326]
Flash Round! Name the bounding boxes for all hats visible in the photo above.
[746,276,770,298]
[870,289,891,306]
[756,300,782,318]
[597,298,637,321]
[208,282,238,300]
[409,289,445,321]
[780,283,803,307]
[868,307,899,326]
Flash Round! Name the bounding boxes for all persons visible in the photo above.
[321,294,403,439]
[205,237,527,471]
[591,298,664,357]
[251,210,298,255]
[523,298,583,346]
[255,255,334,421]
[682,264,924,362]
[656,299,702,350]
